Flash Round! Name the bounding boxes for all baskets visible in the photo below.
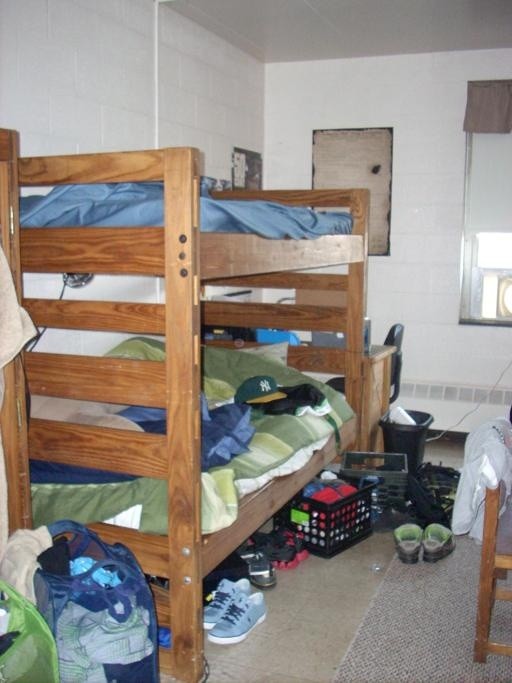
[278,469,378,557]
[342,451,408,501]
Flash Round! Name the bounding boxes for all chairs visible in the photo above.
[382,324,404,404]
[473,417,512,665]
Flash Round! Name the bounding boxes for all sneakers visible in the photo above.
[208,591,267,645]
[424,523,457,562]
[202,578,251,629]
[394,522,422,564]
[253,527,307,569]
[243,546,275,589]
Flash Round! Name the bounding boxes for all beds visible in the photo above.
[1,128,372,681]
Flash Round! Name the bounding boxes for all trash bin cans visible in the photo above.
[378,409,433,473]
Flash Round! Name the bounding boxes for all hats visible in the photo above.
[234,375,288,405]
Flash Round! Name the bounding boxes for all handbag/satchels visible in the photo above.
[30,519,158,683]
[0,577,58,683]
[409,463,460,526]
